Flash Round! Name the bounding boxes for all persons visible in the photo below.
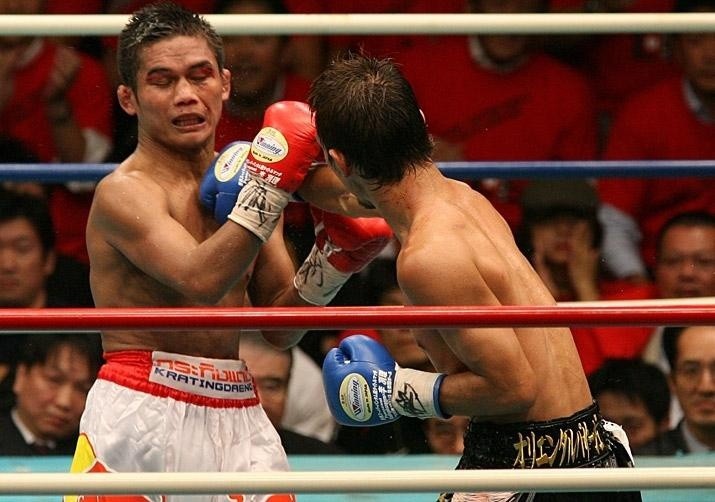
[196,49,644,502]
[61,2,396,502]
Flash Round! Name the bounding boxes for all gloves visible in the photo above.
[322,332,452,427]
[293,197,394,307]
[199,101,321,243]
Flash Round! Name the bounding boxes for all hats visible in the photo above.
[521,175,602,213]
